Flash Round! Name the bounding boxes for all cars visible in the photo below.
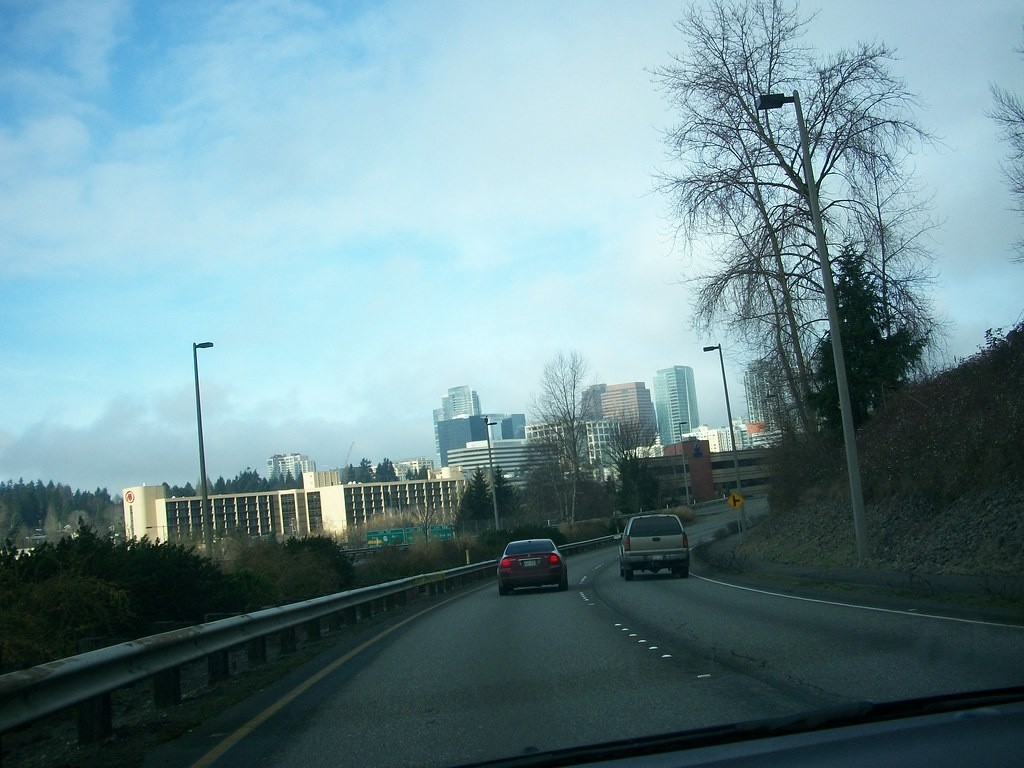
[496,538,569,595]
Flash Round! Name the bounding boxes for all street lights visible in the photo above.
[483,415,501,532]
[753,90,871,566]
[703,344,749,544]
[191,341,216,562]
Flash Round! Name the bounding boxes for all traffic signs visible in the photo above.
[429,525,453,542]
[406,526,426,544]
[387,527,408,546]
[367,530,389,548]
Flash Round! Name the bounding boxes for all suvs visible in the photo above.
[613,512,690,581]
